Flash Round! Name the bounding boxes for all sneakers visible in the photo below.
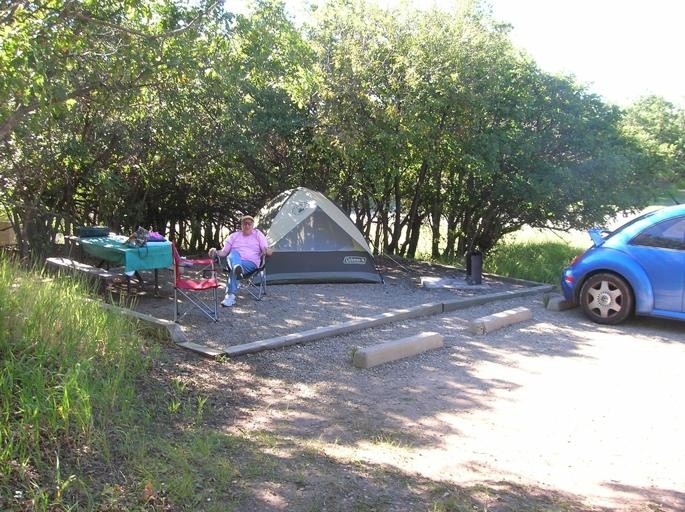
[221,293,236,306]
[234,264,243,280]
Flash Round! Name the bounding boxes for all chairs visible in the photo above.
[224,255,266,301]
[172,240,220,324]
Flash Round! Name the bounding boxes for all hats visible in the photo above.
[242,216,254,221]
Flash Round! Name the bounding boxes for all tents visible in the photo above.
[245,186,383,287]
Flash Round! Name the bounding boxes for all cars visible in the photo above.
[561,204,685,325]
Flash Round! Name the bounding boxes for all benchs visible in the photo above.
[46,257,125,304]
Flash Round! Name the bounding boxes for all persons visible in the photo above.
[208,215,273,306]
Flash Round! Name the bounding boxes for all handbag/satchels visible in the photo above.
[128,226,147,247]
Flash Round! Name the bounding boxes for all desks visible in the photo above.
[64,235,172,294]
